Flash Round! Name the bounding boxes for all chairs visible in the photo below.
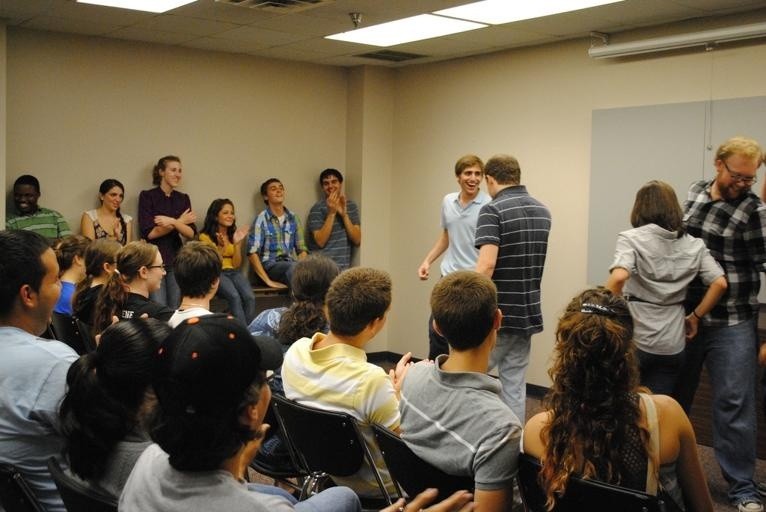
[0,457,117,512]
[39,310,98,358]
[240,386,664,512]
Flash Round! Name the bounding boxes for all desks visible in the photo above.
[212,282,291,304]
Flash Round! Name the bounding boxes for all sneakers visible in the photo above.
[739,498,764,512]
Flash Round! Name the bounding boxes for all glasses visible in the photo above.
[147,264,165,269]
[720,157,757,186]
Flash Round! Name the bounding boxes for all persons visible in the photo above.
[246,178,310,289]
[606,179,728,394]
[474,154,552,428]
[80,178,133,247]
[522,284,714,512]
[306,168,360,269]
[138,156,197,309]
[197,197,257,328]
[417,154,493,361]
[0,227,477,510]
[6,176,71,238]
[400,270,523,510]
[671,136,766,511]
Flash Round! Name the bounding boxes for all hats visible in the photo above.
[158,312,283,414]
